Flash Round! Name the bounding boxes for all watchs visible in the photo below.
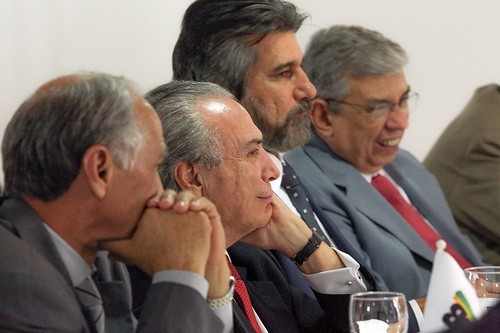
[290,229,322,267]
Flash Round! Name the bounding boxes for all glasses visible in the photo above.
[318,91,421,119]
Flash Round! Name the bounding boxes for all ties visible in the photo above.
[77,278,105,333]
[226,256,262,333]
[282,162,335,248]
[371,173,473,268]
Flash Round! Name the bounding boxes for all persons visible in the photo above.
[282,24,500,304]
[144,80,373,333]
[172,0,427,333]
[421,83,500,266]
[0,69,237,333]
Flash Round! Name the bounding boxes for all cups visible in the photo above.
[464,266,500,300]
[349,291,409,333]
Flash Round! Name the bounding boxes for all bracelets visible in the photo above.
[207,276,237,310]
